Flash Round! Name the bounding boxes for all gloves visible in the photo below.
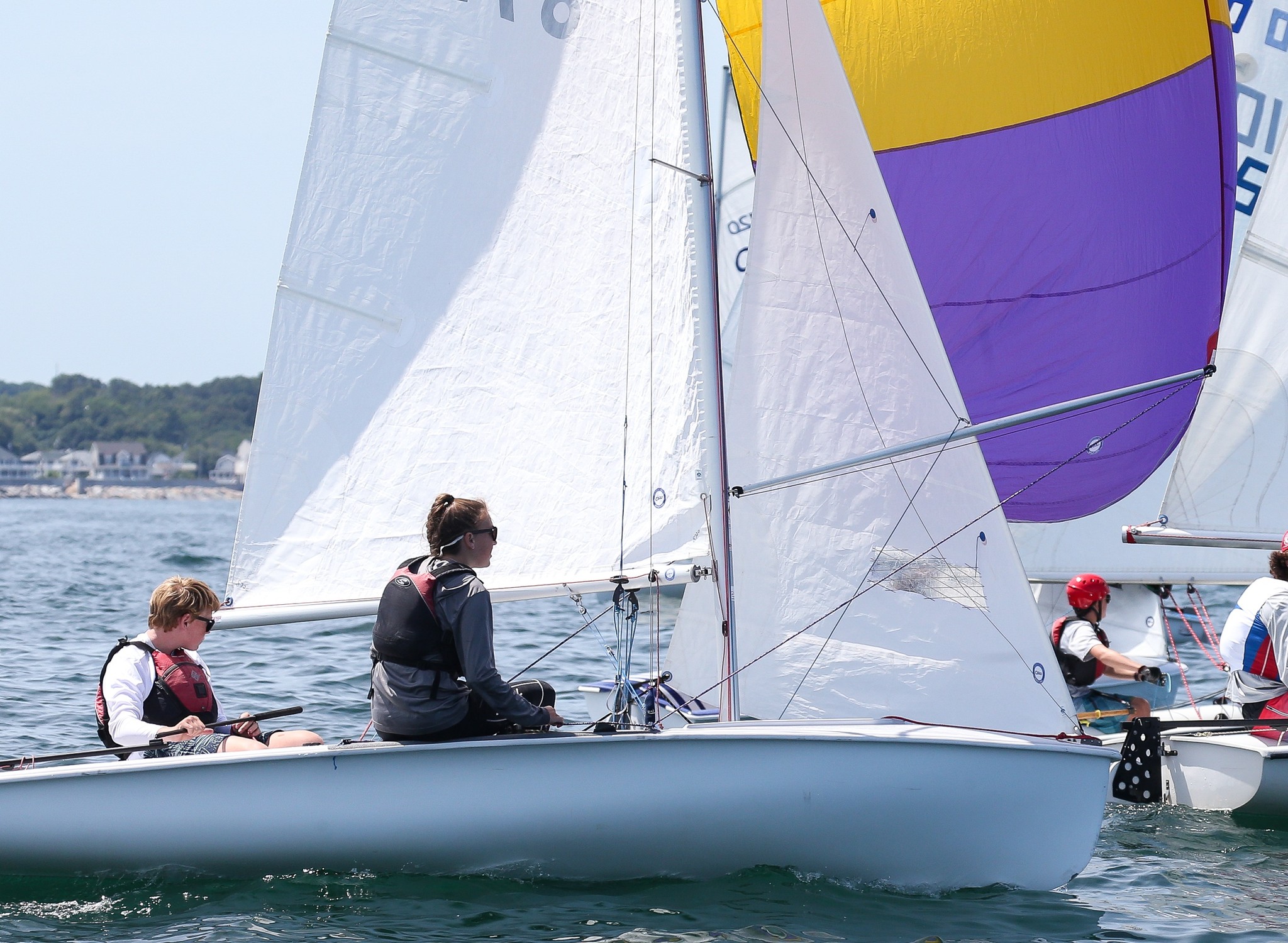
[1134,665,1166,687]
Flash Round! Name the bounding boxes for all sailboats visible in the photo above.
[1001,3,1288,820]
[1,1,1122,892]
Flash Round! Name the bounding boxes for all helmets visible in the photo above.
[1067,573,1111,609]
[1281,530,1288,552]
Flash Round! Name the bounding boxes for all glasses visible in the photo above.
[1104,594,1111,604]
[464,525,499,542]
[186,614,216,632]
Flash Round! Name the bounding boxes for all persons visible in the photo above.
[1049,574,1166,733]
[1220,530,1288,742]
[103,574,322,754]
[368,494,565,740]
